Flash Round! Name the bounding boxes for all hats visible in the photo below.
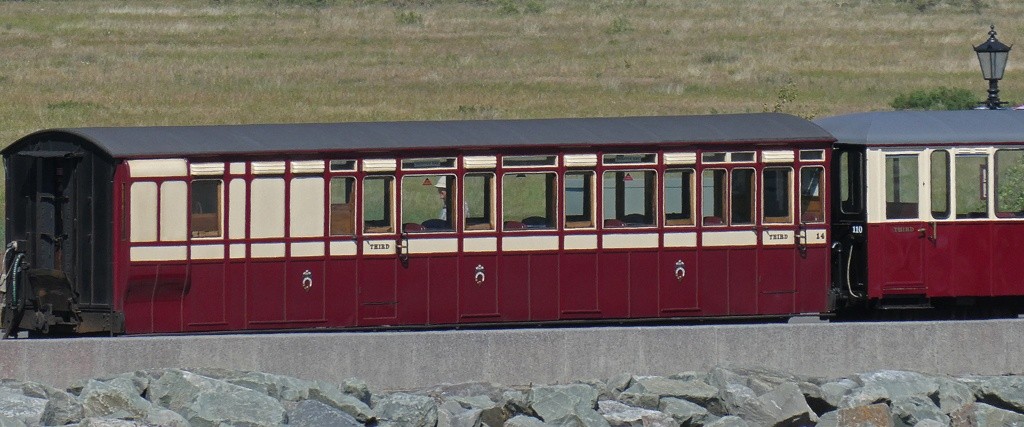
[434,176,446,188]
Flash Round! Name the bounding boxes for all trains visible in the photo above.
[1,107,1024,338]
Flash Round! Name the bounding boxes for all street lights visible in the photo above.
[971,23,1015,109]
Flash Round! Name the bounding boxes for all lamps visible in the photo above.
[972,24,1013,110]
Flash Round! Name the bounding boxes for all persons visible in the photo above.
[434,175,470,220]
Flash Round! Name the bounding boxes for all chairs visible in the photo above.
[362,197,821,234]
